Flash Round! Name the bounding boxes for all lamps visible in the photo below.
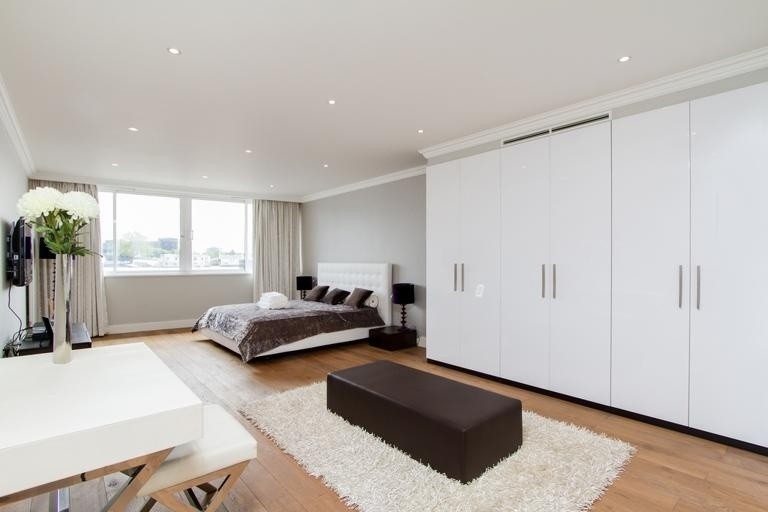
[390,281,415,332]
[296,276,318,298]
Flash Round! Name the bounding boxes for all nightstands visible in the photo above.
[368,325,416,351]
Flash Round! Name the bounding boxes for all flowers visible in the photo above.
[17,185,101,304]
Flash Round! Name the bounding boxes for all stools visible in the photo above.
[140,403,257,511]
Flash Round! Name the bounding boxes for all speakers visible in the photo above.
[39,237,56,259]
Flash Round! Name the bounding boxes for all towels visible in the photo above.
[257,291,289,309]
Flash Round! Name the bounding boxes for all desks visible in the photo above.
[0,341,201,511]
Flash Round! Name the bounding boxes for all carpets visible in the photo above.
[239,376,638,511]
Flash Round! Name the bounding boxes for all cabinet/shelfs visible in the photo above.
[499,115,610,408]
[609,84,768,448]
[426,147,498,382]
[16,319,92,355]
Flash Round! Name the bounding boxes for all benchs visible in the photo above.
[325,359,520,486]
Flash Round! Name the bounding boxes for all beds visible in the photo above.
[189,262,393,362]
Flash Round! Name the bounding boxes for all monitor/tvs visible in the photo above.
[6,216,33,287]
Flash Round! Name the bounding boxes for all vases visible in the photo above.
[53,252,69,363]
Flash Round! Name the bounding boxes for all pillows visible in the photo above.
[321,287,351,305]
[345,287,374,308]
[302,285,329,302]
[343,292,379,308]
